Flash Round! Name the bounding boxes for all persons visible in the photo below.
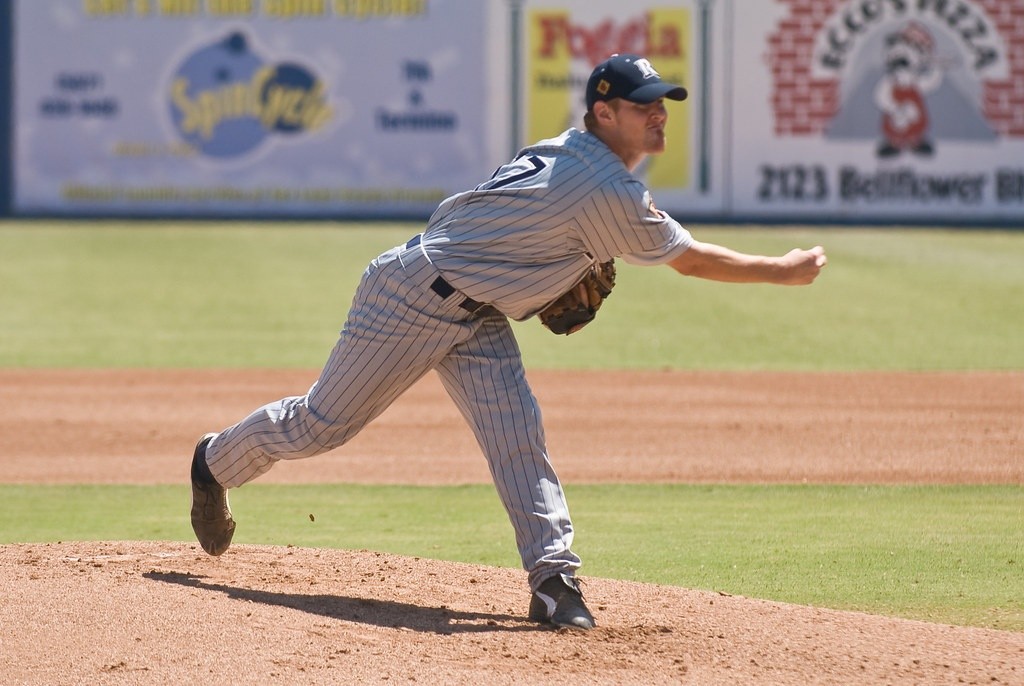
[190,56,827,631]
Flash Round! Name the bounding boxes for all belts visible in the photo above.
[406,235,493,317]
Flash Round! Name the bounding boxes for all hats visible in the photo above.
[586,55,688,112]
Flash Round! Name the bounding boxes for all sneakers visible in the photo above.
[191,433,236,556]
[528,573,596,631]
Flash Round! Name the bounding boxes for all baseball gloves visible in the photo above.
[537,258,616,336]
